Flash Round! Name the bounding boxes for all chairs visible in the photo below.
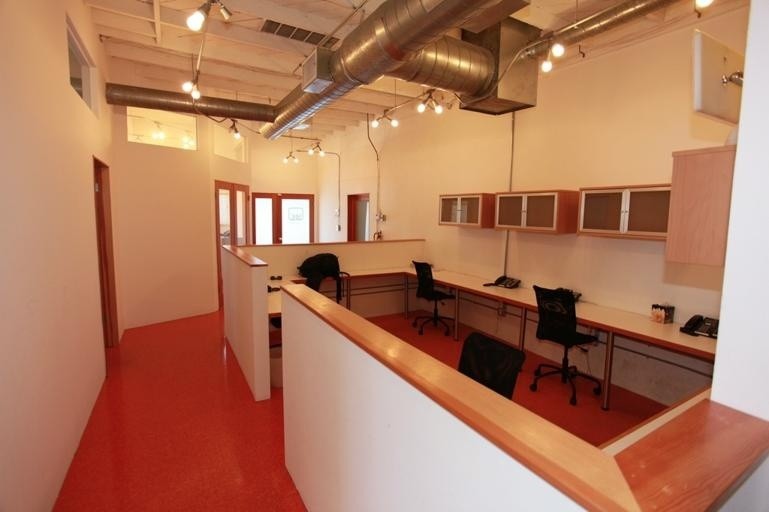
[410,260,454,338]
[530,285,601,405]
[458,332,526,398]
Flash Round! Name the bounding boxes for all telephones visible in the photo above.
[679,314,719,339]
[493,275,521,289]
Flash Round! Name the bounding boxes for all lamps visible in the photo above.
[283,150,300,164]
[541,37,565,75]
[372,108,399,128]
[308,141,325,156]
[227,119,241,141]
[186,1,232,33]
[417,91,444,114]
[182,79,200,100]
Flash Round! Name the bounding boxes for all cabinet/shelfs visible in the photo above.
[577,184,671,241]
[437,194,495,230]
[665,146,735,271]
[493,191,579,236]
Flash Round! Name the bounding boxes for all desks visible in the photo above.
[267,267,717,412]
